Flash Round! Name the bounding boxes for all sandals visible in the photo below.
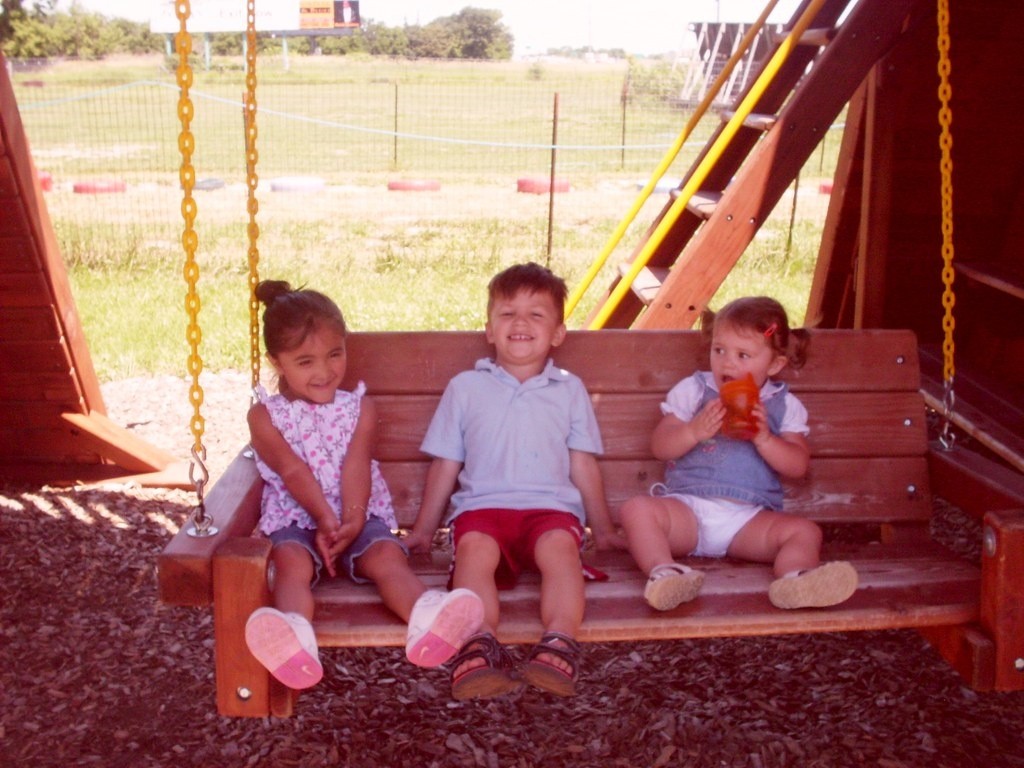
[521,631,582,696]
[450,633,522,700]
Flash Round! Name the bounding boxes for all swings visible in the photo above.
[150,0,1024,702]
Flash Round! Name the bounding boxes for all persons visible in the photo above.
[617,296,859,610]
[403,261,628,700]
[244,280,485,689]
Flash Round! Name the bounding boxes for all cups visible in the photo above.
[720,372,760,441]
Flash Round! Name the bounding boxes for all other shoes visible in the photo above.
[645,563,704,612]
[767,560,858,608]
[244,607,323,689]
[404,588,482,667]
